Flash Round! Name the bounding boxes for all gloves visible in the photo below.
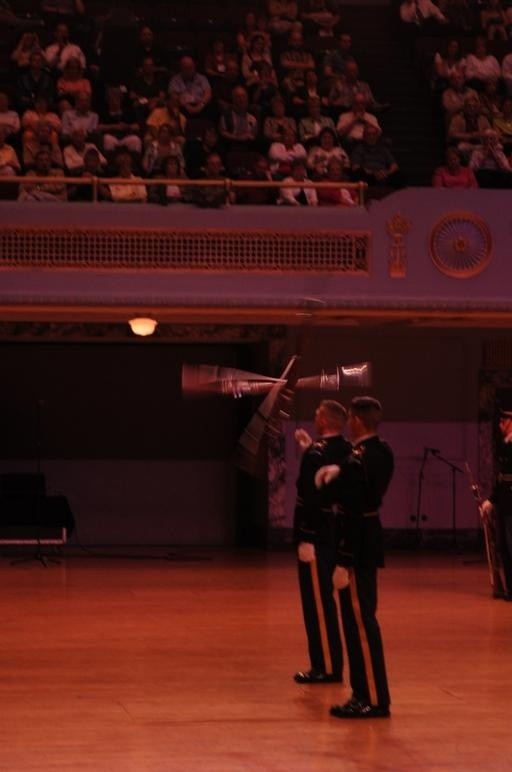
[298,541,316,562]
[295,428,312,449]
[315,464,340,489]
[332,565,349,590]
[479,499,493,517]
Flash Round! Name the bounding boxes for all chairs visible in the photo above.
[0,472,75,566]
[91,0,264,139]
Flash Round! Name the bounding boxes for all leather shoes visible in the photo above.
[293,667,344,683]
[329,695,391,719]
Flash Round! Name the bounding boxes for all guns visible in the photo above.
[465,462,500,588]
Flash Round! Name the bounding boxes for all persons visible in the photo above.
[399,1,446,30]
[431,2,512,190]
[314,395,394,717]
[477,410,512,600]
[291,400,345,683]
[0,1,400,209]
